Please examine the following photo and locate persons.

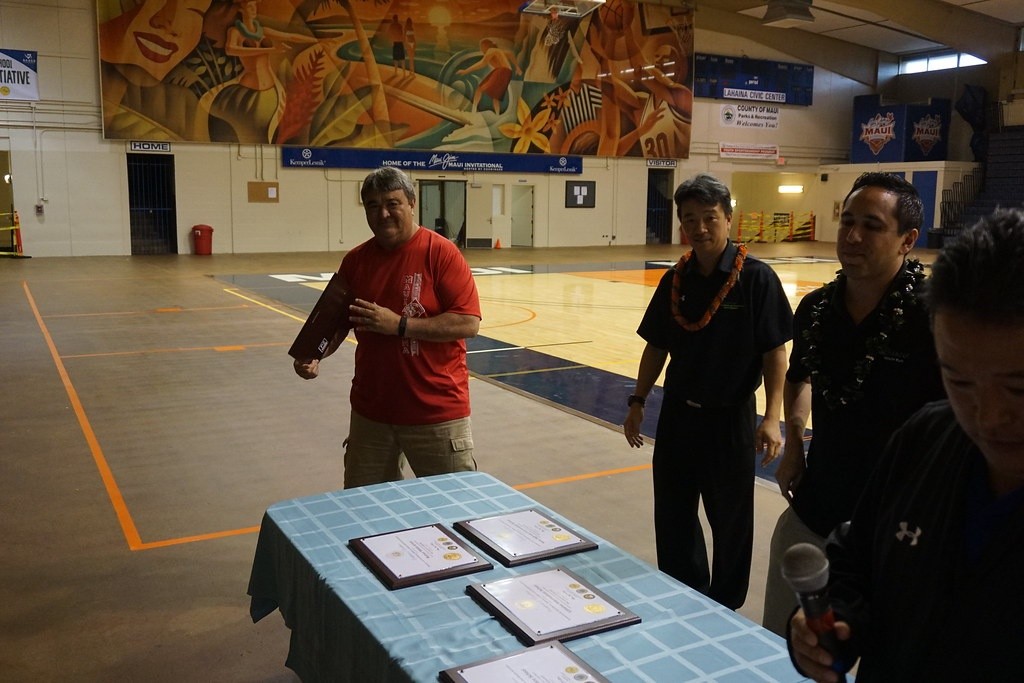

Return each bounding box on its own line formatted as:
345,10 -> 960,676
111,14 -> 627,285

784,207 -> 1024,683
622,175 -> 794,612
293,162 -> 482,488
763,171 -> 946,634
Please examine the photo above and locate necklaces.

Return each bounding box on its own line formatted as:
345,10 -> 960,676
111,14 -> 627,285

671,242 -> 747,331
802,259 -> 926,409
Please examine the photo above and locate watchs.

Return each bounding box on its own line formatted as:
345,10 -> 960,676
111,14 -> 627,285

628,393 -> 645,408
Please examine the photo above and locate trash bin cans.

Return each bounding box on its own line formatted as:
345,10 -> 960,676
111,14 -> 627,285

192,224 -> 213,255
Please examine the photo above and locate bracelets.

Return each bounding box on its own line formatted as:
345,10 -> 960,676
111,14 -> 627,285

399,315 -> 407,337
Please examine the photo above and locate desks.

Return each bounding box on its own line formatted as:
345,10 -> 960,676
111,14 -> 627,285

246,469 -> 856,683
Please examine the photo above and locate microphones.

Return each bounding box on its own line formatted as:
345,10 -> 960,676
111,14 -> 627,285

781,542 -> 847,683
